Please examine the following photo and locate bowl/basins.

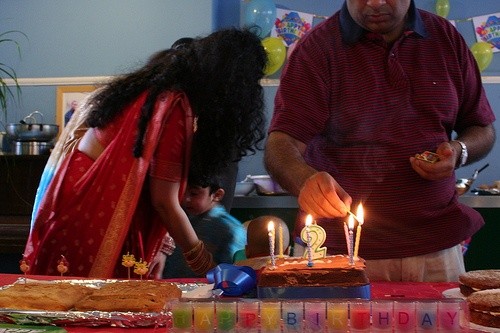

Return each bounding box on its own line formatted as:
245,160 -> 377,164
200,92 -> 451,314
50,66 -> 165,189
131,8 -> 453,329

250,175 -> 287,195
234,182 -> 257,196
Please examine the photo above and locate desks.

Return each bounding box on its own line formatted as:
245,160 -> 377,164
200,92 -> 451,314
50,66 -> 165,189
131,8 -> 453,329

0,272 -> 486,333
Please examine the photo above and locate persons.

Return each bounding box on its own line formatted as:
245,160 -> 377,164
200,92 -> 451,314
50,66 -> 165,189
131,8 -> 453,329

264,0 -> 496,281
64,100 -> 79,127
19,28 -> 264,278
163,138 -> 252,278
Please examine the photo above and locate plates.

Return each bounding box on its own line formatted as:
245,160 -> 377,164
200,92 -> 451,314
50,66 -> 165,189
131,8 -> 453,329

471,188 -> 500,196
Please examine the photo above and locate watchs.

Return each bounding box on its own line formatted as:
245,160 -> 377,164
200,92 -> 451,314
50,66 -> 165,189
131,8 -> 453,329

452,140 -> 468,167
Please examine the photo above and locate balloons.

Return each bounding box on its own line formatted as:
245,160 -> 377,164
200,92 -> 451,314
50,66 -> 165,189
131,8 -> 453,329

241,0 -> 494,77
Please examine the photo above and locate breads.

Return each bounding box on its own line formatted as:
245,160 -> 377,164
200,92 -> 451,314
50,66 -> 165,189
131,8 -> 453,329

75,280 -> 183,313
0,281 -> 95,311
458,268 -> 500,328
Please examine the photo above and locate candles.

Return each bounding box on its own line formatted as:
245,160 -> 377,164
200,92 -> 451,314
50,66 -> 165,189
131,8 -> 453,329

344,201 -> 366,265
268,220 -> 284,267
305,214 -> 314,262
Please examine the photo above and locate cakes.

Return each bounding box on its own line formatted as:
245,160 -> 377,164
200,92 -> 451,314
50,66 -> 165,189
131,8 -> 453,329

256,253 -> 371,301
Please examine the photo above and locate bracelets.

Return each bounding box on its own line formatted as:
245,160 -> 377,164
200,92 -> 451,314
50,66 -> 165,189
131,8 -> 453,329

182,241 -> 213,275
159,234 -> 176,256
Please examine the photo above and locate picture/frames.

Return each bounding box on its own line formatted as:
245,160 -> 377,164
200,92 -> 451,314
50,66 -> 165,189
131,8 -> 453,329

58,84 -> 102,139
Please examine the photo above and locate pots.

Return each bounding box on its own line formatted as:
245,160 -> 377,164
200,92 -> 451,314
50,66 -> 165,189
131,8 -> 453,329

455,169 -> 478,195
6,111 -> 59,141
